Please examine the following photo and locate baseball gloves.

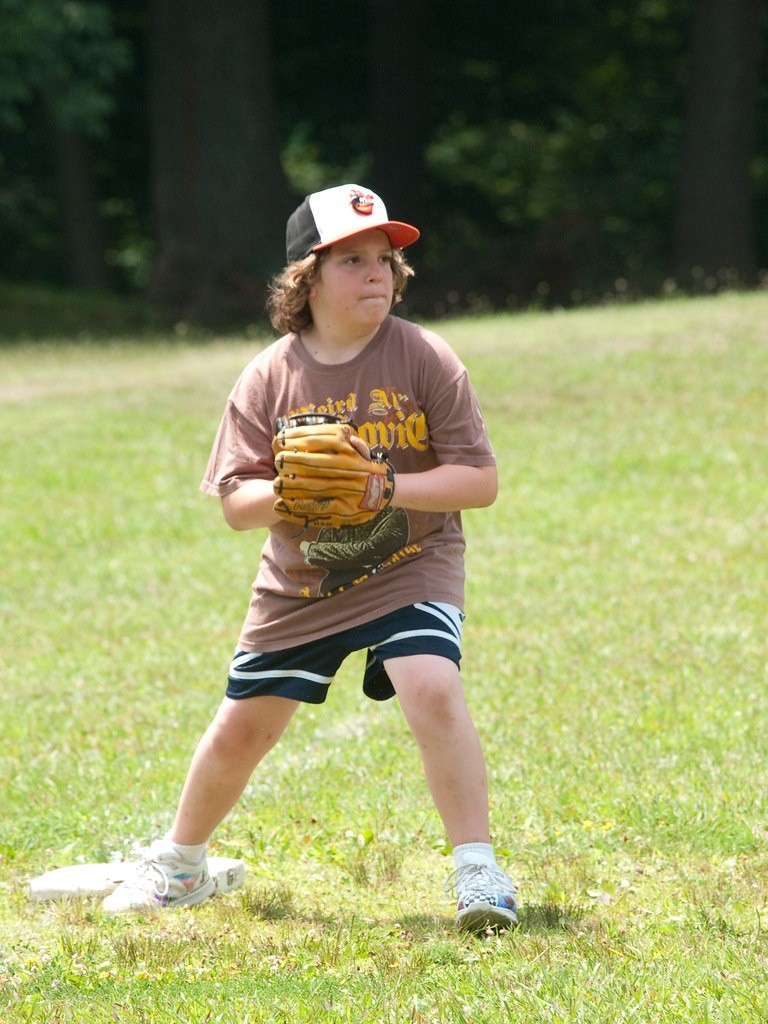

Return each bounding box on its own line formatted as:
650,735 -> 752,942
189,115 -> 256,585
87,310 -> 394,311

270,420 -> 395,530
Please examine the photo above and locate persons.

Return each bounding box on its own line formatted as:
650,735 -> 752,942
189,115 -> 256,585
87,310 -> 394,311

103,184 -> 518,933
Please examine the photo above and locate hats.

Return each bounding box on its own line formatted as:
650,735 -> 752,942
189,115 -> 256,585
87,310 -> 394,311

285,183 -> 421,265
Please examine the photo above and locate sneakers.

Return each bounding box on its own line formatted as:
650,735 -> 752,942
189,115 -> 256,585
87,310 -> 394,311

443,851 -> 518,929
104,841 -> 214,913
